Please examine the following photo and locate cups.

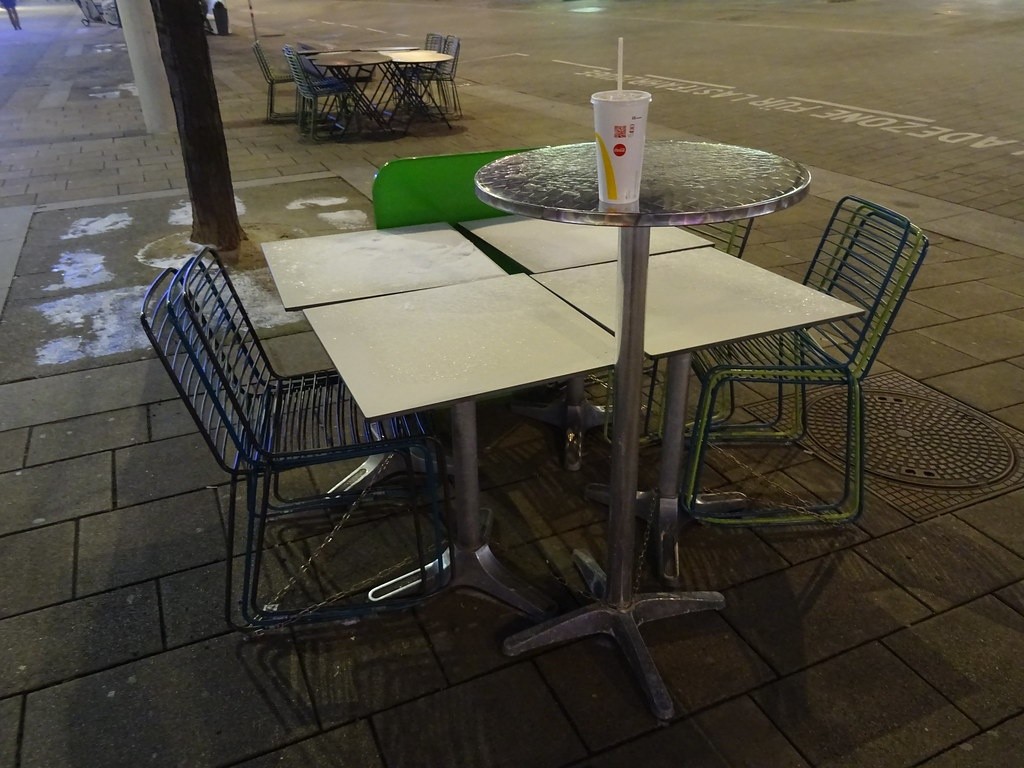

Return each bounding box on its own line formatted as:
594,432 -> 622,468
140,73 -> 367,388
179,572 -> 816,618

591,89 -> 651,203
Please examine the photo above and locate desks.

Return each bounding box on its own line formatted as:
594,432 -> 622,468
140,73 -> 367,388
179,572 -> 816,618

378,50 -> 438,124
474,136 -> 813,723
301,271 -> 649,637
363,46 -> 420,120
259,223 -> 512,518
312,52 -> 398,141
461,205 -> 717,493
304,51 -> 380,136
529,247 -> 866,599
388,52 -> 456,134
296,47 -> 367,120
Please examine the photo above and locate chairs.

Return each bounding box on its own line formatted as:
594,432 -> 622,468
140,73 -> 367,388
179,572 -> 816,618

581,169 -> 930,524
392,31 -> 463,120
251,41 -> 360,141
140,246 -> 456,632
